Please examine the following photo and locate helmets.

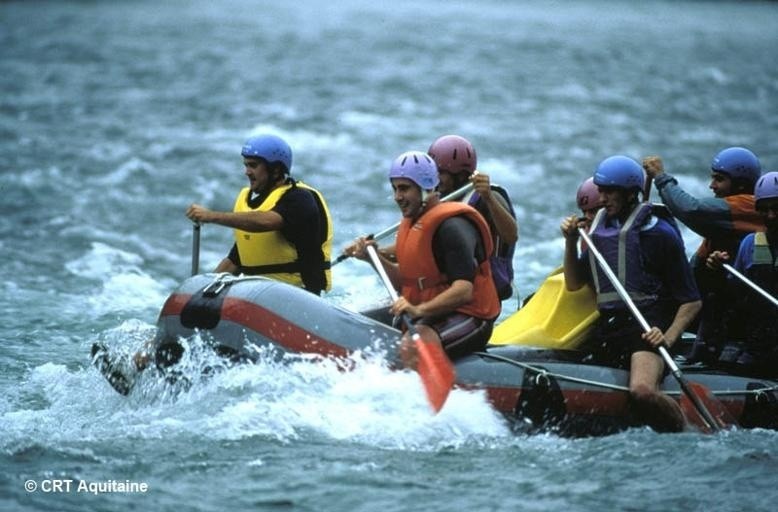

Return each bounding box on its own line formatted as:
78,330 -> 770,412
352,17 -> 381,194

428,134 -> 477,174
711,146 -> 761,183
389,151 -> 442,191
753,173 -> 777,212
576,154 -> 647,211
241,134 -> 291,176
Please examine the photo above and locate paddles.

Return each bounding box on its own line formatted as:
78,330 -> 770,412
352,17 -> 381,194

366,242 -> 456,412
577,226 -> 739,434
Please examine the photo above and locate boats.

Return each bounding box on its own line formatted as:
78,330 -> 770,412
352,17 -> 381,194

155,272 -> 777,437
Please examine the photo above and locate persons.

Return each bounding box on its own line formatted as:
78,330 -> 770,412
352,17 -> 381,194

560,155 -> 702,432
522,177 -> 605,308
643,147 -> 767,334
697,172 -> 778,382
353,151 -> 501,374
91,134 -> 332,394
349,135 -> 518,328
